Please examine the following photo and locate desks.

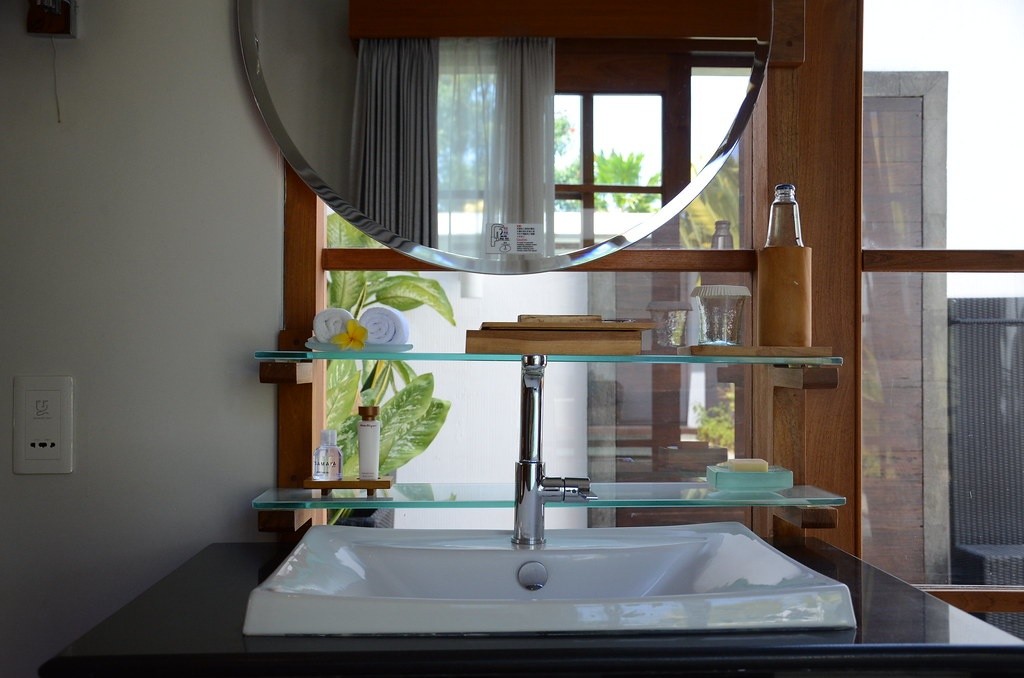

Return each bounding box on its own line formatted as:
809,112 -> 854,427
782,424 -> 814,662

34,544 -> 1024,678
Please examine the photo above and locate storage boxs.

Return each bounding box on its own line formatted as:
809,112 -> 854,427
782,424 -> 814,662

463,330 -> 647,354
706,465 -> 797,490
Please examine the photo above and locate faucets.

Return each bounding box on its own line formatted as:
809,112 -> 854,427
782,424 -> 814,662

510,353 -> 599,546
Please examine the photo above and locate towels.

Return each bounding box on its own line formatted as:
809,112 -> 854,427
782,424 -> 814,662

358,307 -> 406,345
307,307 -> 356,343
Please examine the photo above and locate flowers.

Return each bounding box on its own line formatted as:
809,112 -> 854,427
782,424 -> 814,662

330,318 -> 370,353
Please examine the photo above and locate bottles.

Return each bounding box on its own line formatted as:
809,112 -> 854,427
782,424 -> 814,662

313,430 -> 343,480
764,184 -> 805,247
712,220 -> 734,250
357,406 -> 380,479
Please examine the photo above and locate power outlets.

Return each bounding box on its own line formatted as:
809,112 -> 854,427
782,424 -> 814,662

10,371 -> 77,475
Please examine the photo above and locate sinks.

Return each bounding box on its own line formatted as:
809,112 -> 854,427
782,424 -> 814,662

243,520 -> 857,636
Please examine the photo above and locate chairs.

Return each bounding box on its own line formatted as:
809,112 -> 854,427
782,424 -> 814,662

947,297 -> 1024,639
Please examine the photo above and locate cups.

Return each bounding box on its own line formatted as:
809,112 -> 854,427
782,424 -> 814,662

646,302 -> 694,347
691,285 -> 752,347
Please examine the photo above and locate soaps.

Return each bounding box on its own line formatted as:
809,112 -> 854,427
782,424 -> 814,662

728,458 -> 769,473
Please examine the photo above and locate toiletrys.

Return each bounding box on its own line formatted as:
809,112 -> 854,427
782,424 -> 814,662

357,405 -> 381,481
312,429 -> 344,483
344,481 -> 344,482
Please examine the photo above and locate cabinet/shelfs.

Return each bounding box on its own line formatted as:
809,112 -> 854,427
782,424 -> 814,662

248,338 -> 849,531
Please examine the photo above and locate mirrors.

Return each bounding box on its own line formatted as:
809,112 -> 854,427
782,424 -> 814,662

233,0 -> 774,275
